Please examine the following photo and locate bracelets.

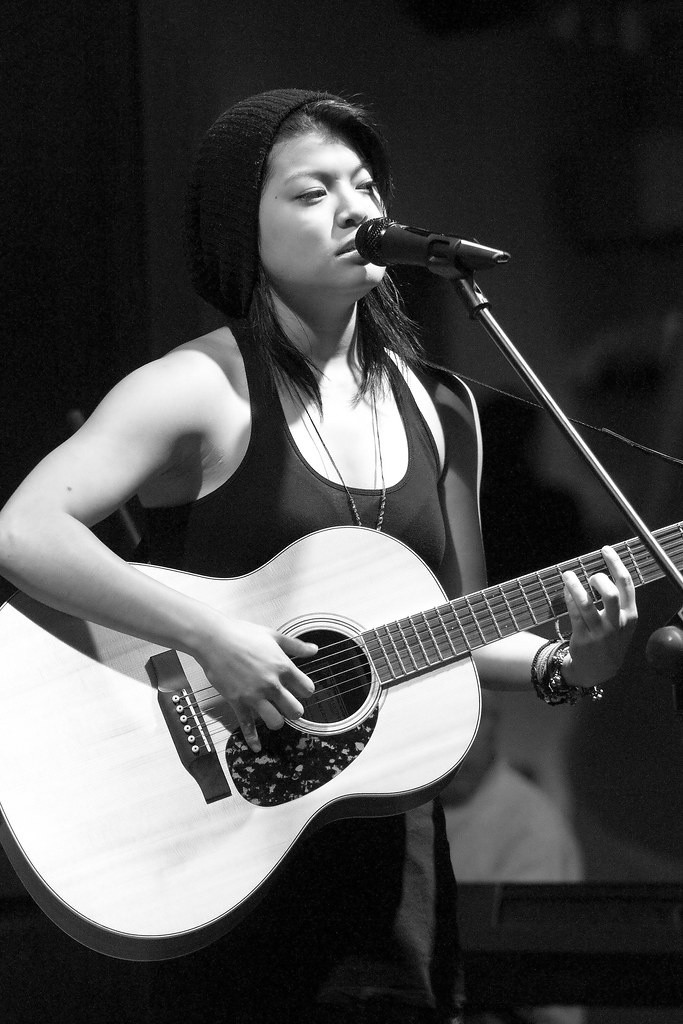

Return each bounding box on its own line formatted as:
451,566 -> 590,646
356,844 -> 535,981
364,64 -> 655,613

530,633 -> 605,707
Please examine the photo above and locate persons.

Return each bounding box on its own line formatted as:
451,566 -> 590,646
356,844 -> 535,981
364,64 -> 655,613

0,86 -> 640,1024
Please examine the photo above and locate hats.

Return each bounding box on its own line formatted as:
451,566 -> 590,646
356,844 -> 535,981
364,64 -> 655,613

179,90 -> 355,319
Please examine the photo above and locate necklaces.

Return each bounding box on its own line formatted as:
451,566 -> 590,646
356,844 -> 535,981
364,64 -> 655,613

288,375 -> 386,533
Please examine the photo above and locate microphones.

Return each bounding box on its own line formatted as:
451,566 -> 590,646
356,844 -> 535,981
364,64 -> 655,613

355,215 -> 510,269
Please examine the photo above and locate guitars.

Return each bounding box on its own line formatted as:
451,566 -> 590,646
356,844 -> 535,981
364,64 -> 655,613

0,523 -> 683,962
